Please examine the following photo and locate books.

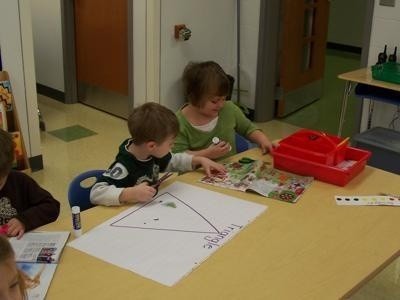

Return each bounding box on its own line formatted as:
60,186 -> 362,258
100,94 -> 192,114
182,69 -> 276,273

9,231 -> 71,300
199,161 -> 315,203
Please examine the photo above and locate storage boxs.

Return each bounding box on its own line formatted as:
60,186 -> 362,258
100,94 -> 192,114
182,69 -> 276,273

352,127 -> 400,172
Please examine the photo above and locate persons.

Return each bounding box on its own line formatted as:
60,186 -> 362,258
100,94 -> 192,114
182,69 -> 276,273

170,61 -> 280,163
90,102 -> 229,206
0,235 -> 29,300
0,128 -> 61,239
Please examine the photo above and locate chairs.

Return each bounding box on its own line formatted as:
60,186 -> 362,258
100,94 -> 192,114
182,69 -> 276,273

67,169 -> 111,213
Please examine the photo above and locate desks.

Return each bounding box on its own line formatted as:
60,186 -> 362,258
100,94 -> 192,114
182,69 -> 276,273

1,138 -> 399,300
334,66 -> 400,138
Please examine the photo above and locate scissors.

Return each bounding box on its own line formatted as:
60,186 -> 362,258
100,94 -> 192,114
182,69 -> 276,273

239,156 -> 273,166
310,128 -> 329,139
141,171 -> 173,198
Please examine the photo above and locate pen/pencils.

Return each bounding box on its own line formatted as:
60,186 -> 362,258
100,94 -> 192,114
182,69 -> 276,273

337,136 -> 350,147
